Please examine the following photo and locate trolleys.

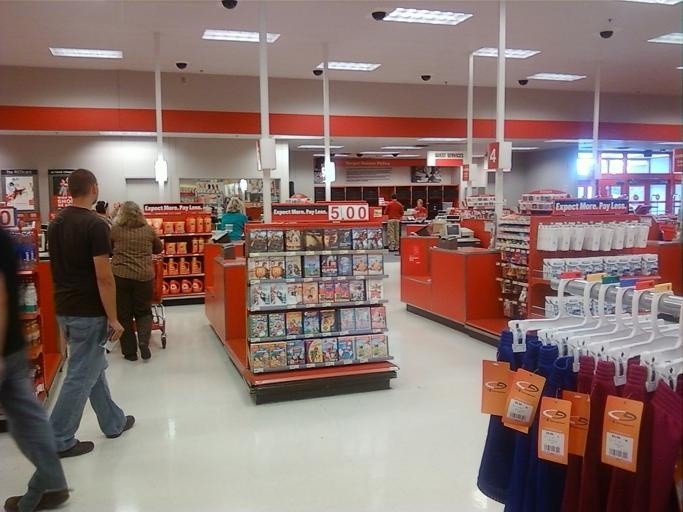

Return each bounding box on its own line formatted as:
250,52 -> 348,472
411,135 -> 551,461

108,252 -> 167,350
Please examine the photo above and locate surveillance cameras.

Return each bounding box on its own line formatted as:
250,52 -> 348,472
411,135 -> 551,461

598,18 -> 614,38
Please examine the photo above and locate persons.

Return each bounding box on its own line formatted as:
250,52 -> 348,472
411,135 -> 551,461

95,200 -> 114,258
412,199 -> 429,219
110,200 -> 164,360
416,166 -> 443,183
0,223 -> 72,512
46,168 -> 135,459
221,197 -> 248,241
384,194 -> 404,252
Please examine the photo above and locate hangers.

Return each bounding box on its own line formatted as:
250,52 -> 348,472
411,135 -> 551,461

508,278 -> 683,392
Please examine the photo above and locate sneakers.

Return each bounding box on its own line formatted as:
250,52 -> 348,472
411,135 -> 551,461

125,354 -> 138,361
139,342 -> 151,359
58,440 -> 95,458
106,415 -> 136,438
4,489 -> 71,510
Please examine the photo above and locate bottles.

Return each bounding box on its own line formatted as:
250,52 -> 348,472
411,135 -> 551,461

185,215 -> 212,255
16,245 -> 47,403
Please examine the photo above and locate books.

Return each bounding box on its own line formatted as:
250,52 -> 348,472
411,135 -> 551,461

247,228 -> 389,370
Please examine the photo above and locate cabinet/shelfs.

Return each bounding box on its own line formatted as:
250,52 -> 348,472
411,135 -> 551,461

134,212 -> 216,300
1,260 -> 68,432
245,224 -> 399,387
495,212 -> 641,318
